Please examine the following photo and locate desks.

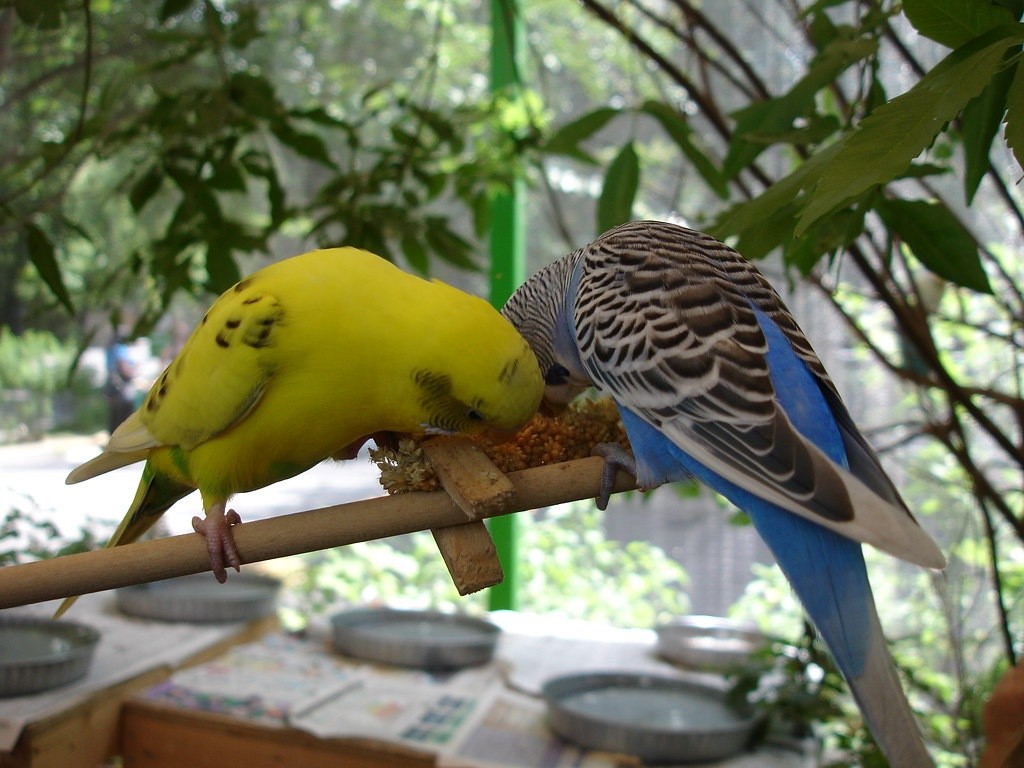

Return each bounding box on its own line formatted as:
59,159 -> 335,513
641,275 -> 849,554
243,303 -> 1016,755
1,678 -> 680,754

1,611 -> 439,768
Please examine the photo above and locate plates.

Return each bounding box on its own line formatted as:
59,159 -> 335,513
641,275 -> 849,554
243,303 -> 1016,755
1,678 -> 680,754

114,574 -> 284,622
538,671 -> 766,761
0,608 -> 100,697
651,618 -> 775,671
331,610 -> 501,670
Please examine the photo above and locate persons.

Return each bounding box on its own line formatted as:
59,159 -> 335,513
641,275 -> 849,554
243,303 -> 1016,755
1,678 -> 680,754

106,340 -> 136,437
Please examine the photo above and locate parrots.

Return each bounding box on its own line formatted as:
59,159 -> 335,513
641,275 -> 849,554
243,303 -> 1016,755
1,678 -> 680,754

56,219 -> 949,767
